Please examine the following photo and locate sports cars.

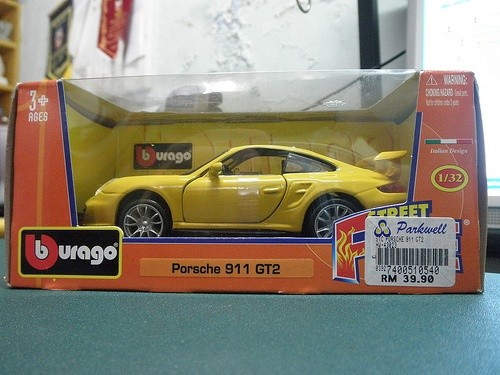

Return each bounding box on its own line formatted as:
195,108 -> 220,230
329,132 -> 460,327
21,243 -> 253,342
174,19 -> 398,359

83,144 -> 408,237
